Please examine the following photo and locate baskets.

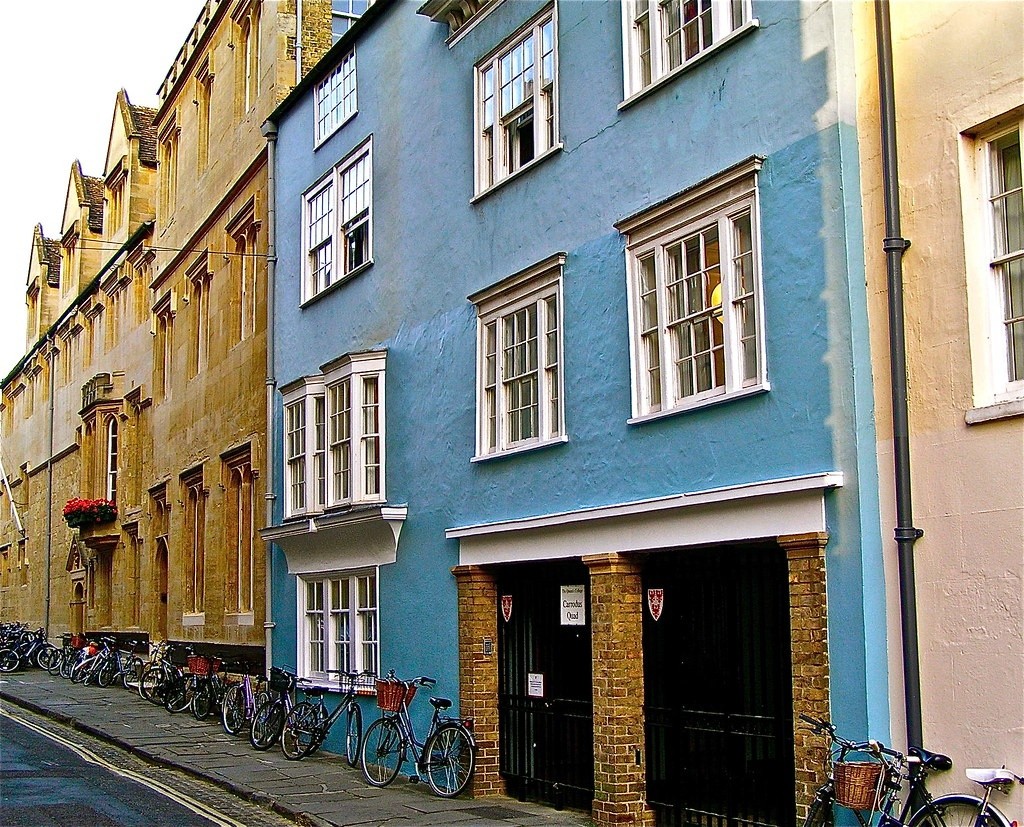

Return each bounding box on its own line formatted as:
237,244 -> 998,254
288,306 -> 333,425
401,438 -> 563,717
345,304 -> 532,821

372,678 -> 418,713
187,655 -> 223,676
71,635 -> 87,649
268,666 -> 297,693
834,758 -> 892,811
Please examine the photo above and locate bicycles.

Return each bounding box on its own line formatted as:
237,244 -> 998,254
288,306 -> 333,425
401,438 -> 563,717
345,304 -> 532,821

0,620 -> 273,735
798,712 -> 1018,827
869,739 -> 1024,827
361,670 -> 479,798
281,669 -> 378,766
248,665 -> 329,755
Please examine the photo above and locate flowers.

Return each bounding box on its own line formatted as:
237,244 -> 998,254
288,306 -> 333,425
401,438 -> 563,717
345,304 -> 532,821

62,497 -> 118,528
